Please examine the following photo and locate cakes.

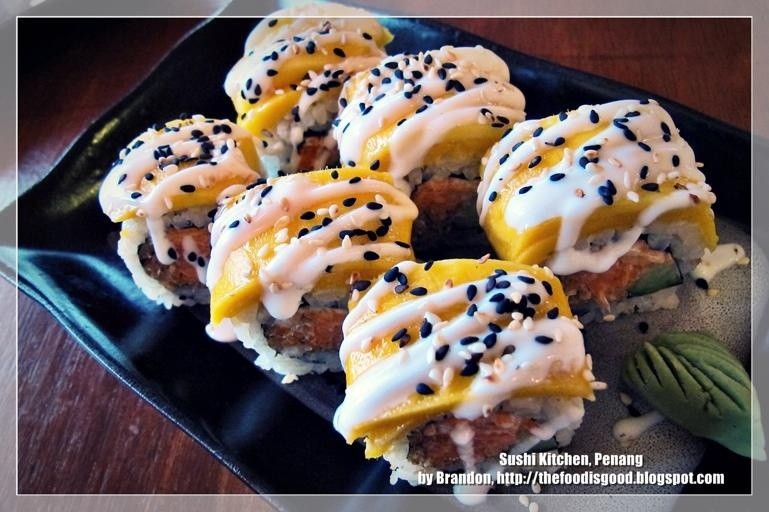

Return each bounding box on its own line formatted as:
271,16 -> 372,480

98,3 -> 718,506
